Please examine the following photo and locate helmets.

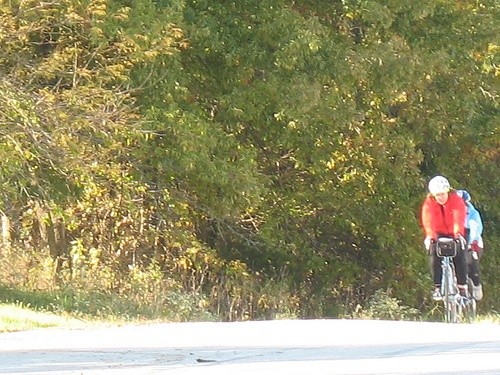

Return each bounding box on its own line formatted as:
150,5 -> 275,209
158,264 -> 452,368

457,190 -> 472,201
428,176 -> 450,194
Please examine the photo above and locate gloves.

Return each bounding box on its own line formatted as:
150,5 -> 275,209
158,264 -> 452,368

455,233 -> 466,250
424,237 -> 433,250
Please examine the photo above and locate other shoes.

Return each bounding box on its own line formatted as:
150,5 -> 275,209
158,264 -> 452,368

470,280 -> 483,301
432,288 -> 442,301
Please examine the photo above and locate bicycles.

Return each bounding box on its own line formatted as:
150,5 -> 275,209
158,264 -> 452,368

427,237 -> 477,324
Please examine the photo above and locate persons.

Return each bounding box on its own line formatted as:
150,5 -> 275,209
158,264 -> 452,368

422,175 -> 469,306
456,190 -> 483,300
